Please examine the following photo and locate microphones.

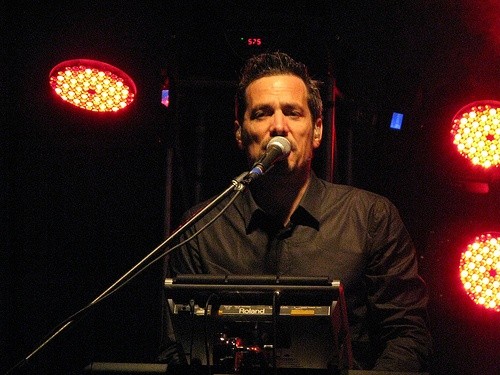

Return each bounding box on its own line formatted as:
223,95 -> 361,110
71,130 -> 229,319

241,136 -> 290,186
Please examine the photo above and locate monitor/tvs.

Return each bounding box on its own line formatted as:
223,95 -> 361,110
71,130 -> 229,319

162,274 -> 354,371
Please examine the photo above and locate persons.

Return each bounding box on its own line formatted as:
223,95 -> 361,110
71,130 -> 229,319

159,49 -> 432,374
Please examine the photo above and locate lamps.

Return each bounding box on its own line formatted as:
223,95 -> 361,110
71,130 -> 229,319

450,99 -> 500,169
49,57 -> 137,113
459,230 -> 499,313
